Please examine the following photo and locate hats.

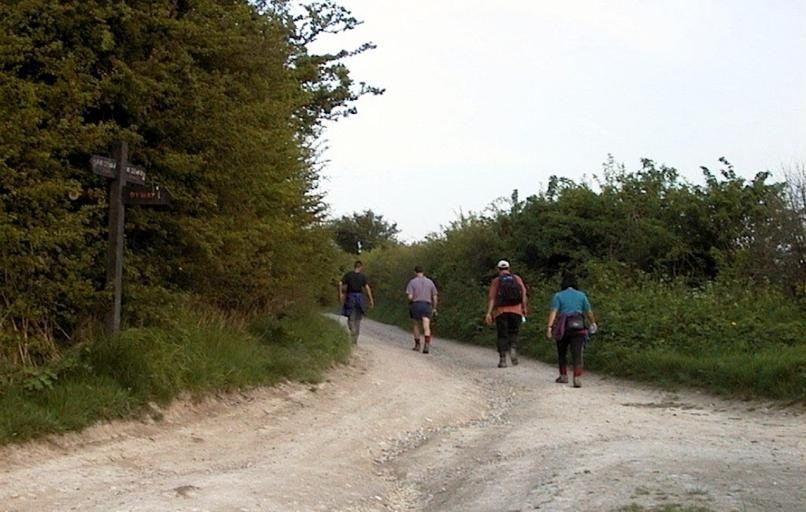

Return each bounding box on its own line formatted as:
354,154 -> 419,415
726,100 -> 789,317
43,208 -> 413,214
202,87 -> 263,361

497,260 -> 510,268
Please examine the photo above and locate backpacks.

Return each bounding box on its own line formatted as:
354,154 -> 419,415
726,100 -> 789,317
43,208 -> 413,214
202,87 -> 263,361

496,274 -> 523,307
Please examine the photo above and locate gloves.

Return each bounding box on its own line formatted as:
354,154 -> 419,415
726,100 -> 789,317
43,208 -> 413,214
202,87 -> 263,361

589,323 -> 597,334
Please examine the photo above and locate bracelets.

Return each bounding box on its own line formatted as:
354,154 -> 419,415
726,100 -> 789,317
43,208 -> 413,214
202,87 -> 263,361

548,325 -> 552,328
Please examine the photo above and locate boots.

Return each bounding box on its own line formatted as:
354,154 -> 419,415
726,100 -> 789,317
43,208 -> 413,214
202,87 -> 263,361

498,350 -> 518,367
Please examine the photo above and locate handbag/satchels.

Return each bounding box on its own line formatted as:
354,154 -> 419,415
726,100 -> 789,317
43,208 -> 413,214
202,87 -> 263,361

567,314 -> 588,330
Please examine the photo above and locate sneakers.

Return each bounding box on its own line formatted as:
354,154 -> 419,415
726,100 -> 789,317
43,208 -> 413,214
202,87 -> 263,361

412,344 -> 429,353
556,375 -> 568,383
574,376 -> 581,387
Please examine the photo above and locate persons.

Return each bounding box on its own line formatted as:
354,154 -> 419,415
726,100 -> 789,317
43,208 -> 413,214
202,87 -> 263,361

546,272 -> 597,387
485,259 -> 527,368
338,260 -> 373,345
406,265 -> 438,353
